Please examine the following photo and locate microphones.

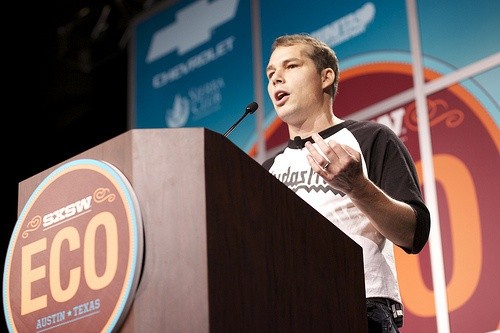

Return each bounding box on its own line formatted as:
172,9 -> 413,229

294,135 -> 302,151
223,102 -> 259,138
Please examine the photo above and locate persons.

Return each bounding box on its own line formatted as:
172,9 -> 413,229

259,33 -> 432,333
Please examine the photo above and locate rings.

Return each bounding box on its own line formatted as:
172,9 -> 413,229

323,161 -> 331,171
